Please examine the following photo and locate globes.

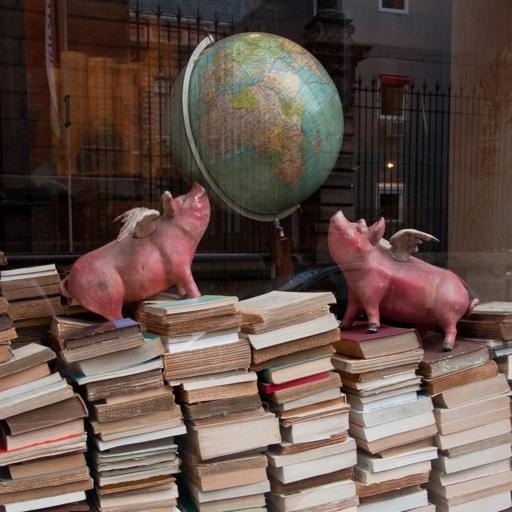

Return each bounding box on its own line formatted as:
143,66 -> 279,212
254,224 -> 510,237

164,30 -> 359,287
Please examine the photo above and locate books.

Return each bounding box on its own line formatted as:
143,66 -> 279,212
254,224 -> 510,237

1,264 -> 94,512
415,300 -> 512,512
133,292 -> 281,512
49,314 -> 188,512
331,319 -> 438,512
238,291 -> 360,512
